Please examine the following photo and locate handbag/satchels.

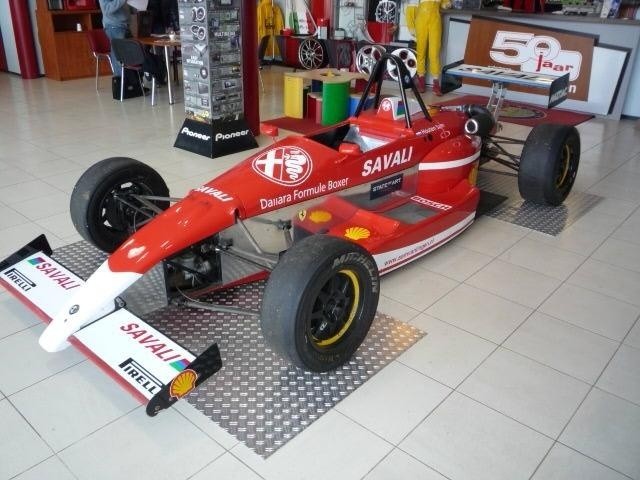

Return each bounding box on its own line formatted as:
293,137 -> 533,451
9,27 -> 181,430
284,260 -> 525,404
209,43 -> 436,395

112,76 -> 140,99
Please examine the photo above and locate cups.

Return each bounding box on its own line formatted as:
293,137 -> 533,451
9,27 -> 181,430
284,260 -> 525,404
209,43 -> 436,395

76,23 -> 83,32
168,34 -> 176,41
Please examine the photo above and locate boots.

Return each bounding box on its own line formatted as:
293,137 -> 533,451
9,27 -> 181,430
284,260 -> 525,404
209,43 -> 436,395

416,76 -> 426,92
432,80 -> 442,97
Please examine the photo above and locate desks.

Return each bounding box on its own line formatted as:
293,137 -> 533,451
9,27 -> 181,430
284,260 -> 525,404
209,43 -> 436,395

284,68 -> 377,126
134,36 -> 181,106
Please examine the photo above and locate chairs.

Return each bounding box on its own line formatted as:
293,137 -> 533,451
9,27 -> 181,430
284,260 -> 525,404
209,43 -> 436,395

111,38 -> 162,106
87,29 -> 115,90
256,33 -> 271,92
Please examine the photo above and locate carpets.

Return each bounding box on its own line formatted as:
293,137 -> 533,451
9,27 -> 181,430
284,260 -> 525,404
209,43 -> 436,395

427,94 -> 595,129
474,159 -> 604,235
51,240 -> 426,459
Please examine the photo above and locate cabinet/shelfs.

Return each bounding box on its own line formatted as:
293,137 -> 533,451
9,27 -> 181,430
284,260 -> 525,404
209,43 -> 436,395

35,0 -> 104,81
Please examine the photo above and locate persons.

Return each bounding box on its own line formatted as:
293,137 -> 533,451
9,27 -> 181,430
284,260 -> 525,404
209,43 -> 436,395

98,0 -> 138,77
404,0 -> 454,96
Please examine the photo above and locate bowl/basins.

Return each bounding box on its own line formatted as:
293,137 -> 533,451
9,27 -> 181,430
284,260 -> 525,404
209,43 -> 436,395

280,28 -> 295,37
